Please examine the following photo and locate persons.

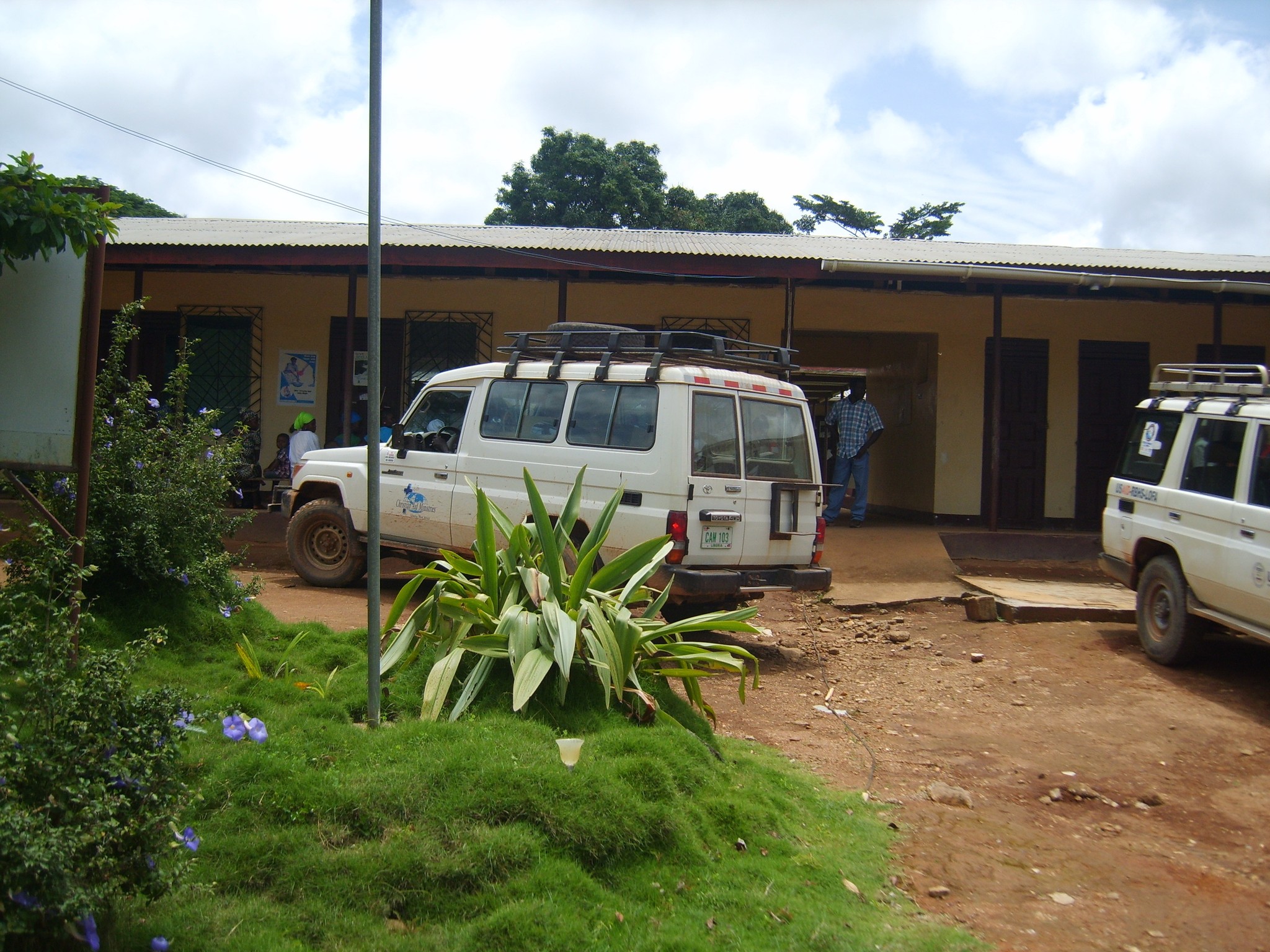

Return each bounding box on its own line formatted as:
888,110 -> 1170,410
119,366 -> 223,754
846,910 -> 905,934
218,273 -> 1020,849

225,405 -> 454,511
818,377 -> 884,528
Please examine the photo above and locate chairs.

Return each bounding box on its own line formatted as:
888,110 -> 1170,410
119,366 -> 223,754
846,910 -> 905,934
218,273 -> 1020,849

1194,440 -> 1241,497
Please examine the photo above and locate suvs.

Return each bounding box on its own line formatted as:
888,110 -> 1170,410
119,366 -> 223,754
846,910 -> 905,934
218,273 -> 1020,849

1097,361 -> 1270,667
266,319 -> 832,638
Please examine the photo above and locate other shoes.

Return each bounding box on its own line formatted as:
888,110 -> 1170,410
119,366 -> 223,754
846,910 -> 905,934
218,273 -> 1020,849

825,520 -> 835,526
230,504 -> 239,508
266,496 -> 271,500
849,520 -> 862,527
253,505 -> 266,509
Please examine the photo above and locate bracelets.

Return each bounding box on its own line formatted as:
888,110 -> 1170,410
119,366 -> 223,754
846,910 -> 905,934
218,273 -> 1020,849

864,444 -> 869,449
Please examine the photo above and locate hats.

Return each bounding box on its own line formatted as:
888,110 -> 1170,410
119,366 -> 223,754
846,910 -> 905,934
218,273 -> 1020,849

427,419 -> 445,432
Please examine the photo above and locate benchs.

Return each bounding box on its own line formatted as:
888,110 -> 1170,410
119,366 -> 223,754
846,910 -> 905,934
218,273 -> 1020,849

228,477 -> 292,486
